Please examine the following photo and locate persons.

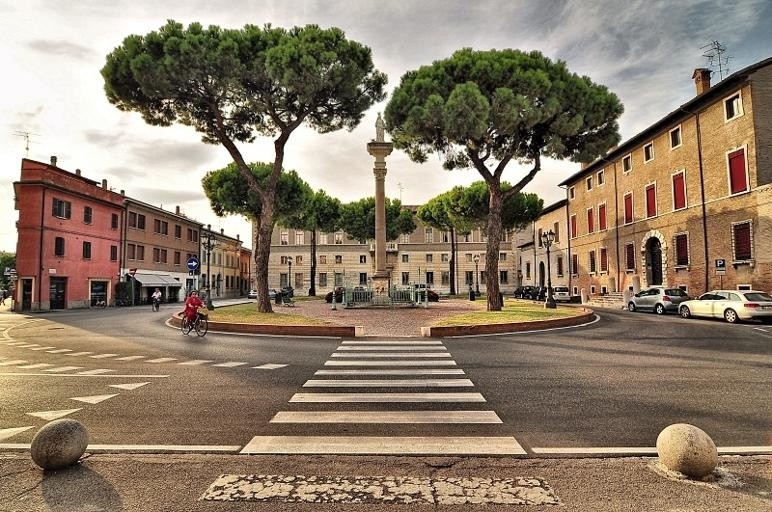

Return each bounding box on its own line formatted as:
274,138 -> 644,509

151,287 -> 161,307
183,290 -> 203,333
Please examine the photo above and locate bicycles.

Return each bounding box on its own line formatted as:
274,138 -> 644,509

181,306 -> 209,337
151,295 -> 160,312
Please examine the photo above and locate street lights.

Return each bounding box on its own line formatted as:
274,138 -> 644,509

200,225 -> 215,310
541,229 -> 556,308
472,251 -> 480,297
287,256 -> 292,287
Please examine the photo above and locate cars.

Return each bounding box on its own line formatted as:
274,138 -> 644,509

514,285 -> 571,302
248,288 -> 278,298
628,287 -> 690,315
677,289 -> 771,322
